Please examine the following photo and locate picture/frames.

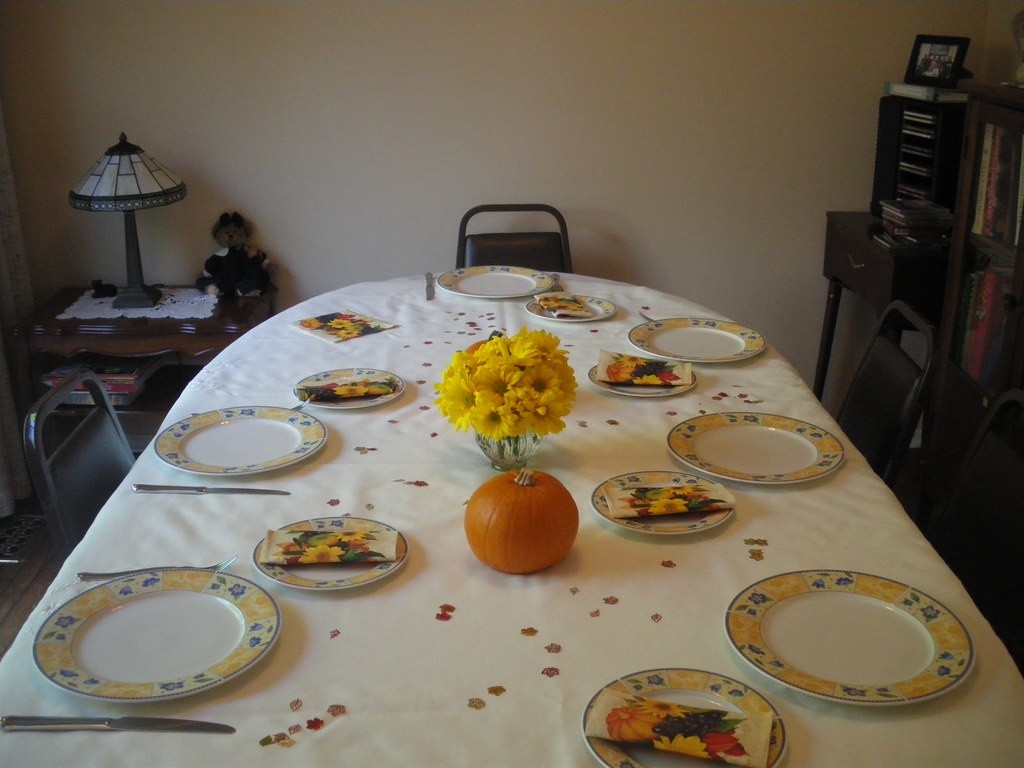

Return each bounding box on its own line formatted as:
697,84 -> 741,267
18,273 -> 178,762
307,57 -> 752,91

904,34 -> 971,89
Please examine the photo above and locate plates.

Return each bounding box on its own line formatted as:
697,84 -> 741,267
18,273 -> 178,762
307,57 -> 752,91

627,318 -> 767,362
725,570 -> 974,705
580,667 -> 788,768
153,406 -> 328,475
588,365 -> 697,397
666,412 -> 845,484
31,567 -> 281,703
293,368 -> 405,408
526,295 -> 615,321
251,517 -> 409,591
436,265 -> 554,298
589,470 -> 734,534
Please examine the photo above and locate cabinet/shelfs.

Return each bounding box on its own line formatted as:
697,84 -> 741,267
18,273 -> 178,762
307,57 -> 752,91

937,76 -> 1023,405
8,285 -> 273,485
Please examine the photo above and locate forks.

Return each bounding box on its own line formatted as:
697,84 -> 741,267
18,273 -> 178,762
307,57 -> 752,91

551,274 -> 563,292
190,394 -> 315,415
638,311 -> 653,321
76,553 -> 237,582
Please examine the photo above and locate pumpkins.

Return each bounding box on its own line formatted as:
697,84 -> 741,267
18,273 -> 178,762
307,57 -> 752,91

465,330 -> 503,357
464,468 -> 580,574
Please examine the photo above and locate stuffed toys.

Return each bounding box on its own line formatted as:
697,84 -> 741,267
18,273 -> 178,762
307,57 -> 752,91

194,212 -> 271,306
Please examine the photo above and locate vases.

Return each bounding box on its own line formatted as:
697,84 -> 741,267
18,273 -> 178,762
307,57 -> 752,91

475,424 -> 543,475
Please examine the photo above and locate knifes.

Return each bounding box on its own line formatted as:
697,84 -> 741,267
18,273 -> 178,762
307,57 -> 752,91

424,272 -> 435,300
0,715 -> 237,734
131,483 -> 291,496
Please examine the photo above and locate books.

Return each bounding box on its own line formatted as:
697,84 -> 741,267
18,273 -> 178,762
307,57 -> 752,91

40,355 -> 163,405
873,124 -> 1024,379
884,82 -> 969,104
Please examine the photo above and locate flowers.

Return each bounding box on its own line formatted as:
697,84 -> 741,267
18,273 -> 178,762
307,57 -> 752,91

436,330 -> 577,441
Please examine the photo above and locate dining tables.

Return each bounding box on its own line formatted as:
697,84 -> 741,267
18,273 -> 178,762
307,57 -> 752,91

0,266 -> 1024,768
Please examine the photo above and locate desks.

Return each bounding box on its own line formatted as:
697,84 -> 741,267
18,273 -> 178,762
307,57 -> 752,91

811,212 -> 951,401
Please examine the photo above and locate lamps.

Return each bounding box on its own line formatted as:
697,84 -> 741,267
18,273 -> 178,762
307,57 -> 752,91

68,132 -> 187,309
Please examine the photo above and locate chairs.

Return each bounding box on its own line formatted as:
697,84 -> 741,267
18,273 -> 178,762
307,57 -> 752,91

22,368 -> 139,564
929,388 -> 1024,652
456,204 -> 573,279
835,299 -> 939,487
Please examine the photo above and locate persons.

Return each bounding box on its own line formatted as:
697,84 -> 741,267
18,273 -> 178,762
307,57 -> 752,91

920,55 -> 954,79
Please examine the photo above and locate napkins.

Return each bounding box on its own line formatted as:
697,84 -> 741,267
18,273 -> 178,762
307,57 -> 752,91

291,309 -> 398,344
533,292 -> 592,318
584,686 -> 774,768
601,482 -> 736,519
259,526 -> 399,563
595,349 -> 692,387
294,378 -> 396,404
